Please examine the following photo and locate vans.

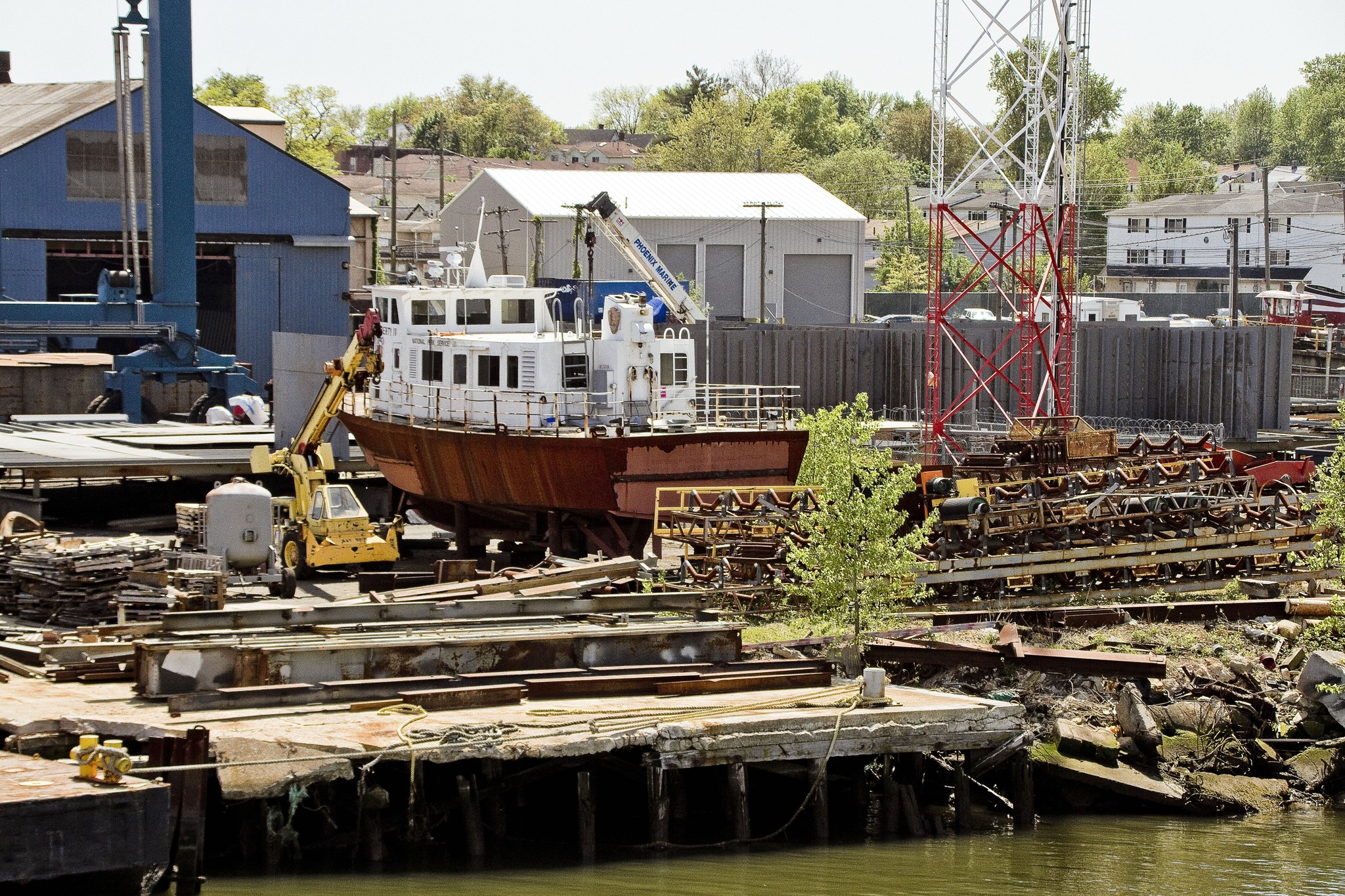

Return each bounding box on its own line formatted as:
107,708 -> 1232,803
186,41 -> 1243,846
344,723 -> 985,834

961,308 -> 1030,322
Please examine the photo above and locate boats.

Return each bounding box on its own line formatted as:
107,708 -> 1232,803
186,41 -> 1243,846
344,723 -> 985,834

337,110 -> 809,519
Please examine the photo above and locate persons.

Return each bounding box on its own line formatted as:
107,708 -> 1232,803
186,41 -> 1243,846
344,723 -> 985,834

264,378 -> 273,423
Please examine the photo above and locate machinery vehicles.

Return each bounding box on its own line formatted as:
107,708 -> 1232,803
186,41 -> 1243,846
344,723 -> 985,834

250,307 -> 405,598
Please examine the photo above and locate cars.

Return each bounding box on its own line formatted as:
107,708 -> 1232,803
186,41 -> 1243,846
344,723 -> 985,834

920,307 -> 938,316
1140,312 -> 1215,327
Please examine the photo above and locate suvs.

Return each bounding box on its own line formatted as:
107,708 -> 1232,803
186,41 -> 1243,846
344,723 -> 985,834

1211,308 -> 1248,326
871,315 -> 927,324
